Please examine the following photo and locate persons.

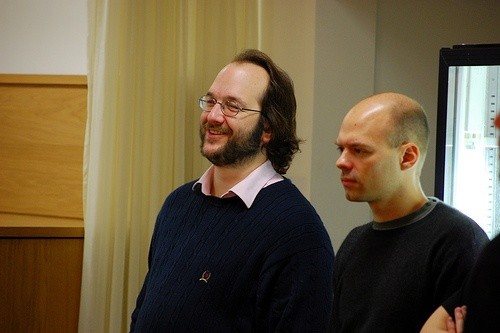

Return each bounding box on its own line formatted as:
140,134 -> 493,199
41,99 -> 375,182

331,93 -> 491,333
418,110 -> 500,333
123,46 -> 340,333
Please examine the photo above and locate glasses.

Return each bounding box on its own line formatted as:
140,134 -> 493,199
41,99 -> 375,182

198,95 -> 264,117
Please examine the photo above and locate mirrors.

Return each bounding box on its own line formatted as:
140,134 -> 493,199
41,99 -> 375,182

433,43 -> 500,241
